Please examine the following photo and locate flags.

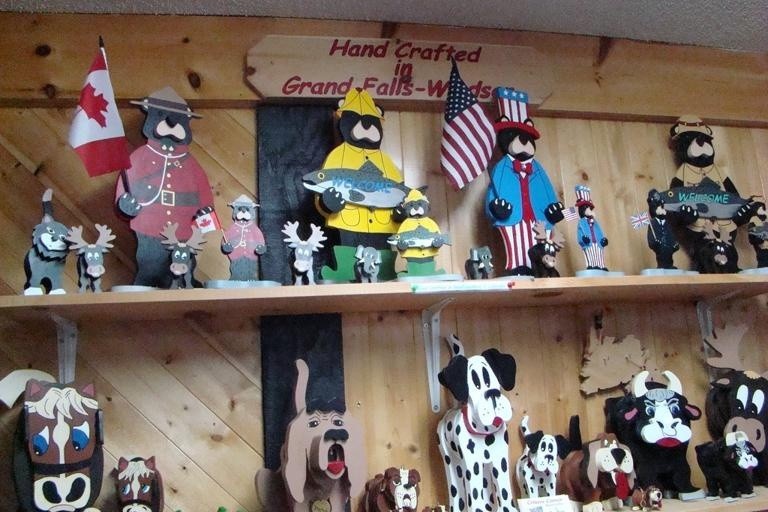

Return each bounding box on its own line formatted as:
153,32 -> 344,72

67,43 -> 132,177
438,60 -> 501,192
